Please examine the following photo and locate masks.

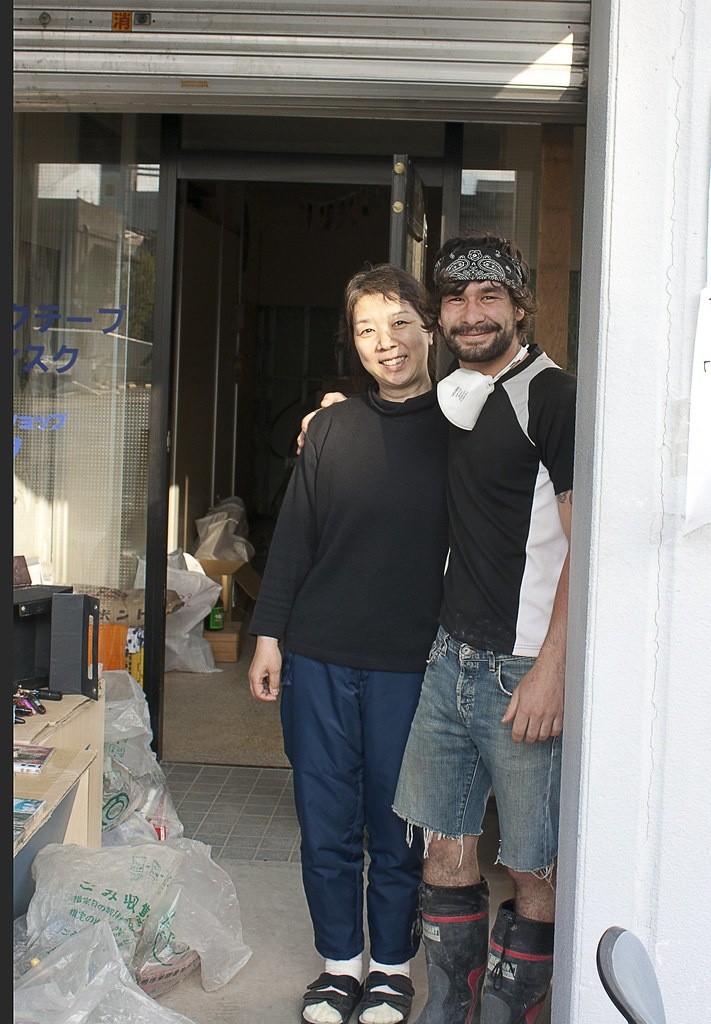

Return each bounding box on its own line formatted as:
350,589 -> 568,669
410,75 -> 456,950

437,344 -> 529,430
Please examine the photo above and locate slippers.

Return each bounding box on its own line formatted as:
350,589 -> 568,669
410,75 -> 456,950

300,972 -> 365,1024
356,970 -> 415,1024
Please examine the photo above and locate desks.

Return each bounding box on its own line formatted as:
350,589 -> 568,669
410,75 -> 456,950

13,680 -> 107,916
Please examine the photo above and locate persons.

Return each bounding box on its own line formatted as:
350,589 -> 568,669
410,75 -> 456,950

297,232 -> 577,1024
248,263 -> 449,1024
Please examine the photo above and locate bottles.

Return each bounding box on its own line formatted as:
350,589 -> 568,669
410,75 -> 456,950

205,596 -> 224,632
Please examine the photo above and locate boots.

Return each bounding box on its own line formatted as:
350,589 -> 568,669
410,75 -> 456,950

413,875 -> 491,1024
479,898 -> 555,1024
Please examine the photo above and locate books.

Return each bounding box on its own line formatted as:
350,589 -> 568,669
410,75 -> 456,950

13,742 -> 56,775
13,796 -> 48,853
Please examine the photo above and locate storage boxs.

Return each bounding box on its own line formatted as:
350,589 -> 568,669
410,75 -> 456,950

203,621 -> 242,663
